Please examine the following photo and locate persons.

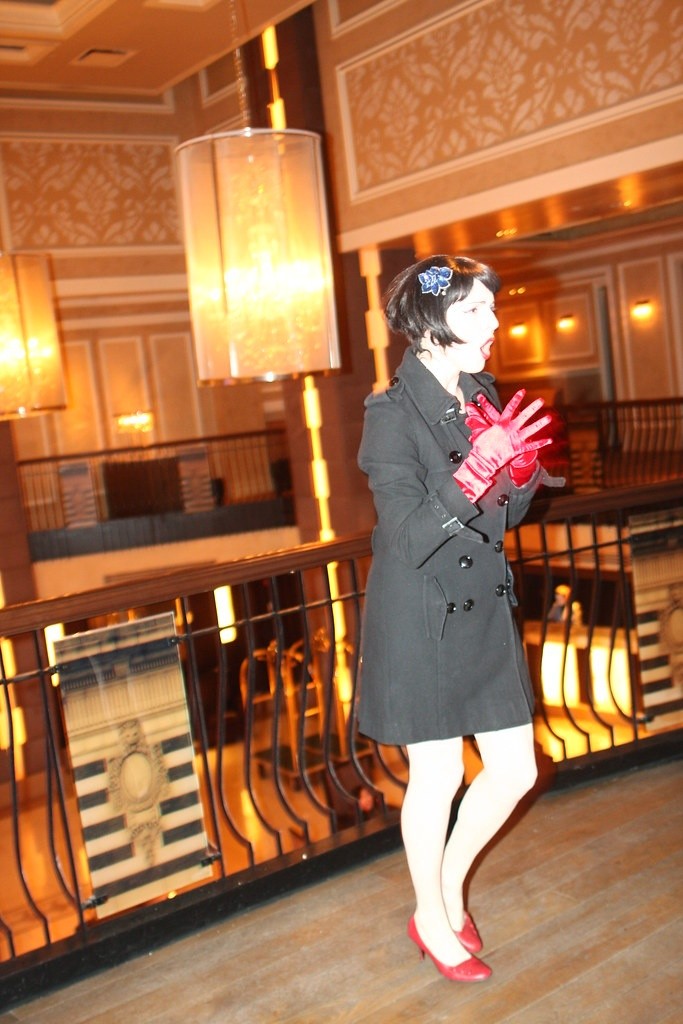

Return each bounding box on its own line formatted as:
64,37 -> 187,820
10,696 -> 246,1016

355,254 -> 554,984
547,584 -> 583,623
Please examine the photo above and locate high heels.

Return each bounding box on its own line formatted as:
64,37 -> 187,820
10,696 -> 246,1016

407,914 -> 491,983
450,910 -> 484,953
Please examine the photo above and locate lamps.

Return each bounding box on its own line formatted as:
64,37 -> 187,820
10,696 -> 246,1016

0,253 -> 68,423
172,0 -> 340,381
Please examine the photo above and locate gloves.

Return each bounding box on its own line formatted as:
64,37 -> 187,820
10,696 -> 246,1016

462,393 -> 538,488
453,389 -> 554,503
